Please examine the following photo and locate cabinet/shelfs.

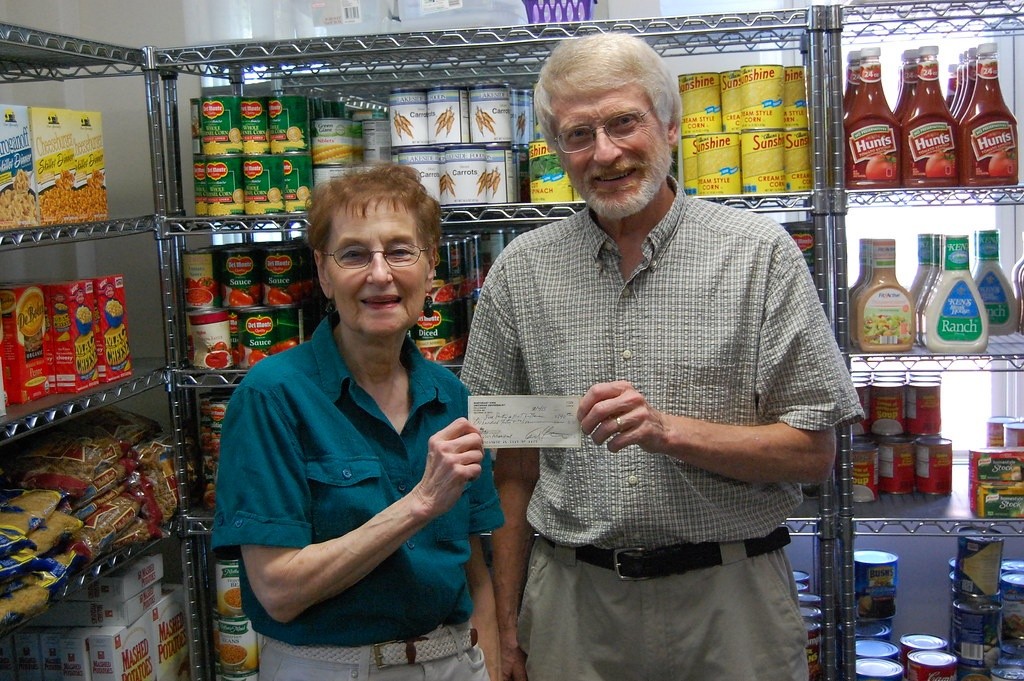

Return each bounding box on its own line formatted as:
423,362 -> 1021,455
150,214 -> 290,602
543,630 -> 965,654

0,17 -> 205,681
830,1 -> 1024,681
155,9 -> 840,681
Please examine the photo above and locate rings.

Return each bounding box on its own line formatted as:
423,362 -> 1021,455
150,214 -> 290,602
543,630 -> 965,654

616,416 -> 620,425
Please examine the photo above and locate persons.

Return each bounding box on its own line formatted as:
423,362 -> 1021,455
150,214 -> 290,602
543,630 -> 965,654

459,34 -> 866,681
212,168 -> 500,681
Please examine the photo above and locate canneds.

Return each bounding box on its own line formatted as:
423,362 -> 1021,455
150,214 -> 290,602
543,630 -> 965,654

780,221 -> 815,281
675,64 -> 812,196
187,80 -> 589,218
793,569 -> 823,681
179,228 -> 536,372
199,386 -> 229,513
850,372 -> 953,503
854,526 -> 1024,681
208,546 -> 262,681
986,416 -> 1024,447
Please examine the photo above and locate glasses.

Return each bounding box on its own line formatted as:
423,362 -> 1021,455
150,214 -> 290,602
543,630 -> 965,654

319,241 -> 431,269
552,104 -> 654,154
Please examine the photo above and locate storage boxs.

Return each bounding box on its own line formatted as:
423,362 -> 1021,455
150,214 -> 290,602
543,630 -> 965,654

0,104 -> 109,231
0,548 -> 189,681
0,275 -> 132,418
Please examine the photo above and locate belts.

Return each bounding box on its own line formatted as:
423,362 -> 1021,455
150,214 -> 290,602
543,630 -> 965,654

264,618 -> 480,670
540,524 -> 792,581
